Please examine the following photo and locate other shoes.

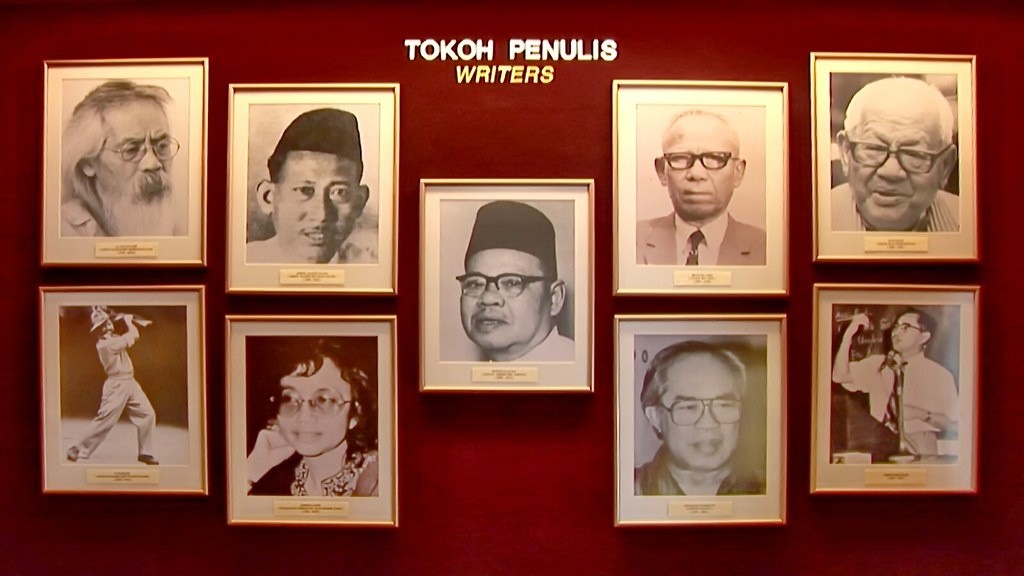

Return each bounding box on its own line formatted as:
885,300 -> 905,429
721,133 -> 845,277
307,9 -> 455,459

138,454 -> 159,465
68,446 -> 79,460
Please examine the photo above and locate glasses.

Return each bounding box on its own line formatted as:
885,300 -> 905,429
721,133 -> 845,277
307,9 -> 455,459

662,150 -> 739,170
456,271 -> 552,298
659,396 -> 742,425
102,137 -> 180,161
892,321 -> 923,334
845,131 -> 951,174
269,386 -> 355,417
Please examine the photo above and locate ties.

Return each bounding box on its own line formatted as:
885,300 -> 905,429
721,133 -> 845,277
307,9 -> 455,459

684,230 -> 706,266
882,358 -> 908,434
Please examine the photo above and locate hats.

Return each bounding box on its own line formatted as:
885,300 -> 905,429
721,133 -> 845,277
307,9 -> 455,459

276,108 -> 361,163
464,201 -> 557,279
90,308 -> 113,332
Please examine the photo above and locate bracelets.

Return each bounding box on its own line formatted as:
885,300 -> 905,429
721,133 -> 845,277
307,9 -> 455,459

247,477 -> 256,488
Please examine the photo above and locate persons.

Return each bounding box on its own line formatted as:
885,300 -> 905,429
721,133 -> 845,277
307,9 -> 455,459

66,305 -> 159,466
455,200 -> 573,362
635,110 -> 767,266
634,341 -> 766,496
832,307 -> 959,456
247,338 -> 378,497
831,75 -> 959,232
60,80 -> 189,237
246,107 -> 379,265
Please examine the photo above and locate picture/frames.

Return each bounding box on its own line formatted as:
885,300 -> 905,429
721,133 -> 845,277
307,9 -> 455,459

41,57 -> 207,266
39,284 -> 208,495
223,316 -> 399,526
224,83 -> 400,296
611,77 -> 789,296
810,51 -> 982,261
809,283 -> 980,494
419,178 -> 595,393
612,311 -> 787,524
